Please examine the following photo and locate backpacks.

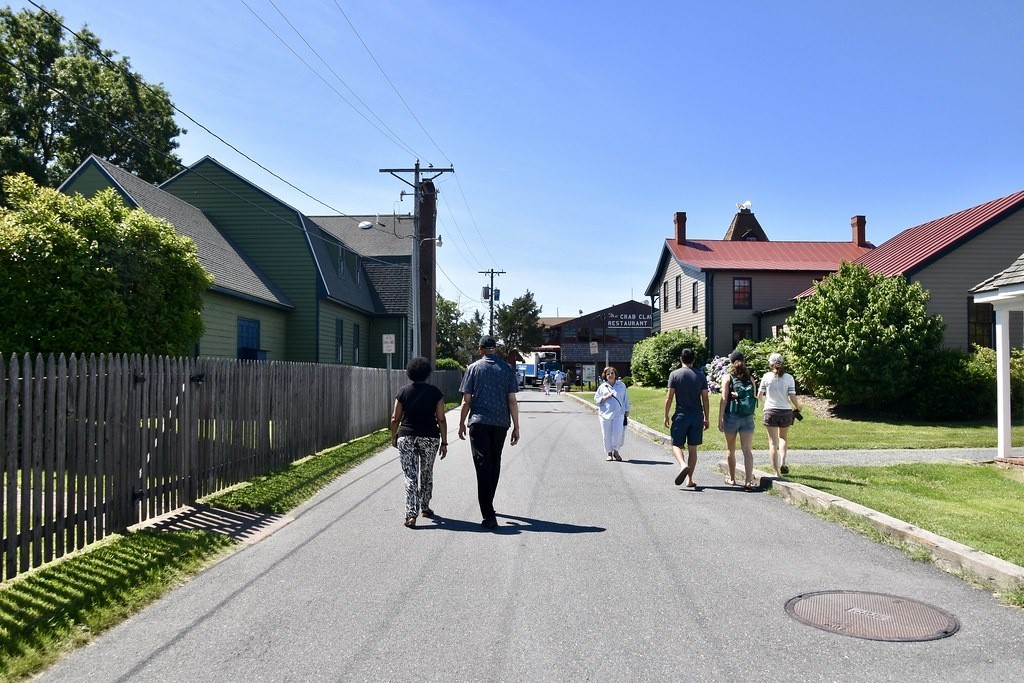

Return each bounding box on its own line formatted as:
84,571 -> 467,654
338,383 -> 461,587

729,372 -> 758,416
546,375 -> 552,383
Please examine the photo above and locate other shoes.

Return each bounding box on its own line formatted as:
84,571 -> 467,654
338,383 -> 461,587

482,519 -> 498,530
606,452 -> 613,461
781,465 -> 789,474
404,517 -> 416,527
613,450 -> 622,461
422,508 -> 434,516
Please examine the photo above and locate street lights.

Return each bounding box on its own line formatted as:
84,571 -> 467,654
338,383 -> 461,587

415,234 -> 444,358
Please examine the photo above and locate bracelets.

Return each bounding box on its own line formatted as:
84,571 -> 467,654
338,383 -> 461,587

441,441 -> 448,446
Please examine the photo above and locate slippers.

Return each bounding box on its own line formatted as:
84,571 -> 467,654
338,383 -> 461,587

686,483 -> 696,487
745,482 -> 751,488
724,478 -> 735,485
675,467 -> 690,485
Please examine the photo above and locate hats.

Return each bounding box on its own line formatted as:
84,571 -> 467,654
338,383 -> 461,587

730,351 -> 744,363
768,353 -> 783,364
479,335 -> 496,349
556,370 -> 560,373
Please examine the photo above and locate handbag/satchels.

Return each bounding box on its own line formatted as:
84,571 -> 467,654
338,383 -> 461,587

623,414 -> 627,426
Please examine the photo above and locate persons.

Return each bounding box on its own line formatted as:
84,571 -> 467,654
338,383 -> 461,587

457,335 -> 520,527
757,353 -> 801,478
543,369 -> 552,395
664,349 -> 710,486
391,357 -> 448,526
554,370 -> 562,396
718,351 -> 758,485
565,370 -> 571,388
594,366 -> 630,460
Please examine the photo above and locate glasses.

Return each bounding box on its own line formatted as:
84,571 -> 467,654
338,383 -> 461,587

606,372 -> 615,375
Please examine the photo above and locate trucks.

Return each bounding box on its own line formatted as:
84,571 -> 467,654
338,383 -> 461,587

516,351 -> 567,388
